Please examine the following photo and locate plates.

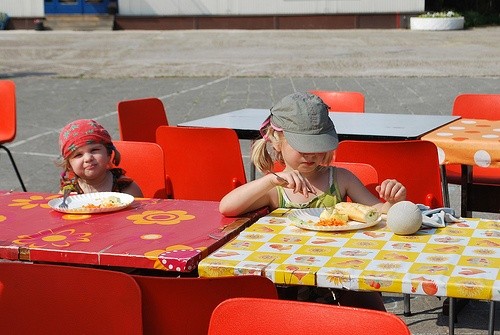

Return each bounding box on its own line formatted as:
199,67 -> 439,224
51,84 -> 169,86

48,192 -> 134,213
285,208 -> 384,231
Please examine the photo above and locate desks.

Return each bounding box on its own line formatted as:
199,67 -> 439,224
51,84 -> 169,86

198,207 -> 500,335
0,189 -> 271,271
177,108 -> 500,206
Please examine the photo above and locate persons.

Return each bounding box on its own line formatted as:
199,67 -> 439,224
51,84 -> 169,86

58,119 -> 145,198
219,92 -> 406,314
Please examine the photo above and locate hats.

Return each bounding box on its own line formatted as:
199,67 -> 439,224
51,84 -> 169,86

269,92 -> 338,154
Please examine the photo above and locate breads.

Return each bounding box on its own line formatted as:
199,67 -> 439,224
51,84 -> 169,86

320,208 -> 348,223
335,201 -> 378,222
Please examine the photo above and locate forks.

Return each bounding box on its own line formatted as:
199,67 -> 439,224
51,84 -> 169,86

58,188 -> 71,209
266,169 -> 329,213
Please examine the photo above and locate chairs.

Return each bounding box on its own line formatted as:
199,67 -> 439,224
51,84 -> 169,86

311,90 -> 500,314
156,126 -> 247,200
0,261 -> 143,335
129,274 -> 278,335
0,78 -> 28,192
210,297 -> 411,335
117,97 -> 169,143
112,140 -> 167,199
